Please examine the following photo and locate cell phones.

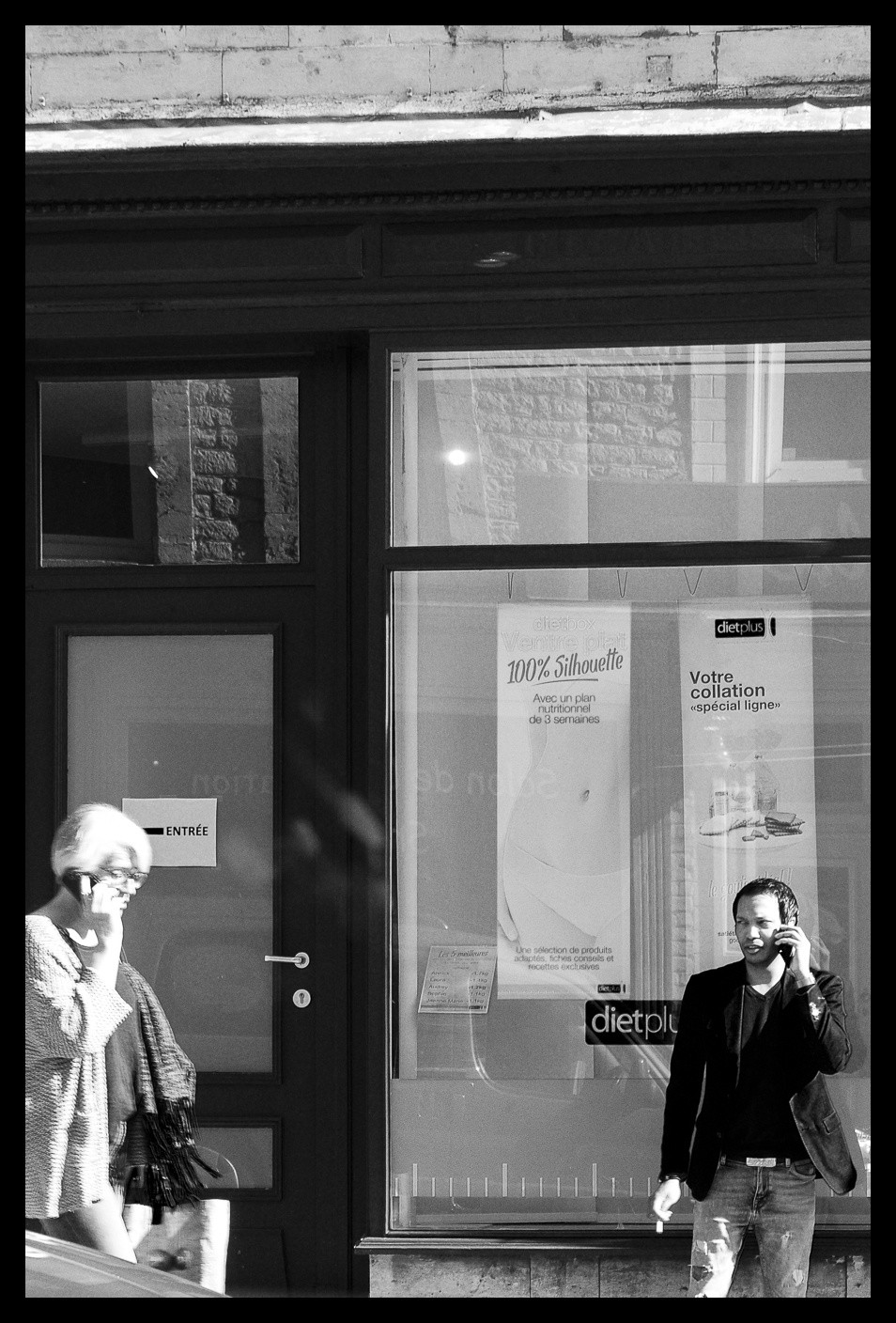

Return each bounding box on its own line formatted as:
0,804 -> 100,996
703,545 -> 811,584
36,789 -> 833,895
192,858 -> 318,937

778,922 -> 797,966
61,867 -> 101,902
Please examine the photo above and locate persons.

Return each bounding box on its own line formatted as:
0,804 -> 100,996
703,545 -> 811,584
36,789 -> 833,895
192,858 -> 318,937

653,877 -> 860,1298
26,803 -> 153,1264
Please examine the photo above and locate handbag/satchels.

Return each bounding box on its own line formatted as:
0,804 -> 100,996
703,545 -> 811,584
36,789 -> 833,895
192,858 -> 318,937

121,1107 -> 232,1294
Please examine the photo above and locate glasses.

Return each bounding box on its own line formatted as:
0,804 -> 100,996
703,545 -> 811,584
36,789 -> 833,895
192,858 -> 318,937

98,867 -> 149,890
